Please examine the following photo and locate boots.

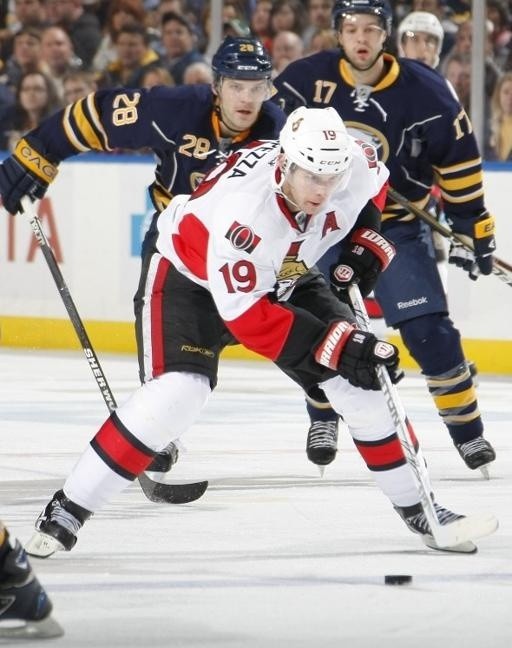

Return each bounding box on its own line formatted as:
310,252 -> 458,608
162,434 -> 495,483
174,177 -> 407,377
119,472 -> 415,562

306,407 -> 344,479
22,489 -> 93,559
0,537 -> 65,638
392,492 -> 469,542
143,438 -> 180,486
446,423 -> 496,481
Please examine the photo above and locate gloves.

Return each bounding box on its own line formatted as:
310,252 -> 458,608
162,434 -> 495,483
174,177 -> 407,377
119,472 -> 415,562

0,129 -> 64,216
328,225 -> 398,305
444,212 -> 498,280
312,319 -> 405,392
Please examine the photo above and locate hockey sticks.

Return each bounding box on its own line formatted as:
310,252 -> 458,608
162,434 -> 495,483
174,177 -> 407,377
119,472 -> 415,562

18,193 -> 208,505
347,280 -> 500,549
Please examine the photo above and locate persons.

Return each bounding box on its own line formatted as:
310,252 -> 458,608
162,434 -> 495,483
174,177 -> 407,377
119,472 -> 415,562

266,0 -> 495,471
1,34 -> 342,466
1,1 -> 512,164
23,108 -> 496,558
1,520 -> 52,625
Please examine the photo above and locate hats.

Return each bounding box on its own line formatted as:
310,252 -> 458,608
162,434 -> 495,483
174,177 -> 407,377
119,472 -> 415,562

160,11 -> 196,32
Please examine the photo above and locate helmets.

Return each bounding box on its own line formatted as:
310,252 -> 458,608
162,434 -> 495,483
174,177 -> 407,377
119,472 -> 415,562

395,10 -> 445,70
278,105 -> 354,193
329,0 -> 393,38
210,33 -> 273,102
